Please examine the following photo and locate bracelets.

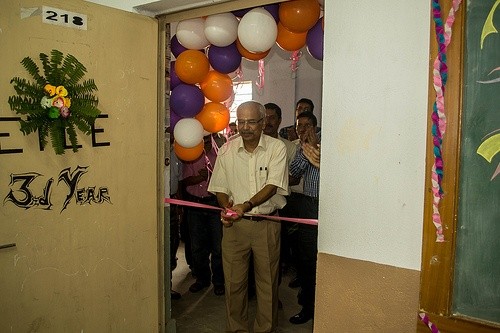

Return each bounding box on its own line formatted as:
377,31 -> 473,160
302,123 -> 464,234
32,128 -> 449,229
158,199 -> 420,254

243,201 -> 253,212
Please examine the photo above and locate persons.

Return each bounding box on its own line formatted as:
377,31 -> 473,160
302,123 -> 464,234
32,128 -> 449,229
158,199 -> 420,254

210,102 -> 287,333
169,96 -> 323,328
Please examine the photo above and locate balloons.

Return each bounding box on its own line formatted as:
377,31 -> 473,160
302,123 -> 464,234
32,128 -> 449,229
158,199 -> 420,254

195,103 -> 230,133
173,119 -> 205,148
166,2 -> 278,116
168,111 -> 183,135
274,20 -> 307,51
307,19 -> 324,62
172,138 -> 205,162
277,1 -> 321,33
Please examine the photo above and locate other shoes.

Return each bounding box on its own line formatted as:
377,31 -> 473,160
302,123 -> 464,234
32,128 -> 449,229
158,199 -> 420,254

189,280 -> 211,292
213,282 -> 225,295
288,277 -> 300,288
171,290 -> 181,300
277,299 -> 283,309
289,308 -> 314,324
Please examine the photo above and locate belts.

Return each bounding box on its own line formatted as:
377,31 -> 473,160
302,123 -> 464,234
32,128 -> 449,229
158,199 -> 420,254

243,210 -> 278,221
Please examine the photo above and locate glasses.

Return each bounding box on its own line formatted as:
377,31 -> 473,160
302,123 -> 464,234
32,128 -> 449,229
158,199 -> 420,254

235,118 -> 263,125
296,107 -> 311,114
